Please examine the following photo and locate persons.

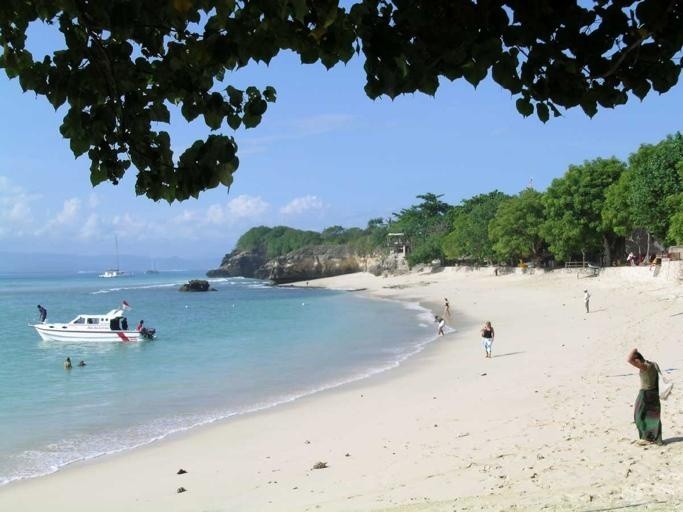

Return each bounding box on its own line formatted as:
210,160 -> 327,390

443,297 -> 449,315
433,316 -> 445,337
627,346 -> 663,448
36,304 -> 47,322
137,320 -> 147,335
121,317 -> 128,330
583,289 -> 590,315
63,356 -> 71,369
78,361 -> 86,367
479,321 -> 494,357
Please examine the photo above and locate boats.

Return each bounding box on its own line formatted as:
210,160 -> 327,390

28,302 -> 157,343
100,269 -> 129,278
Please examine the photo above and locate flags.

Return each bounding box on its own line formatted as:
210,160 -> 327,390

122,299 -> 132,313
